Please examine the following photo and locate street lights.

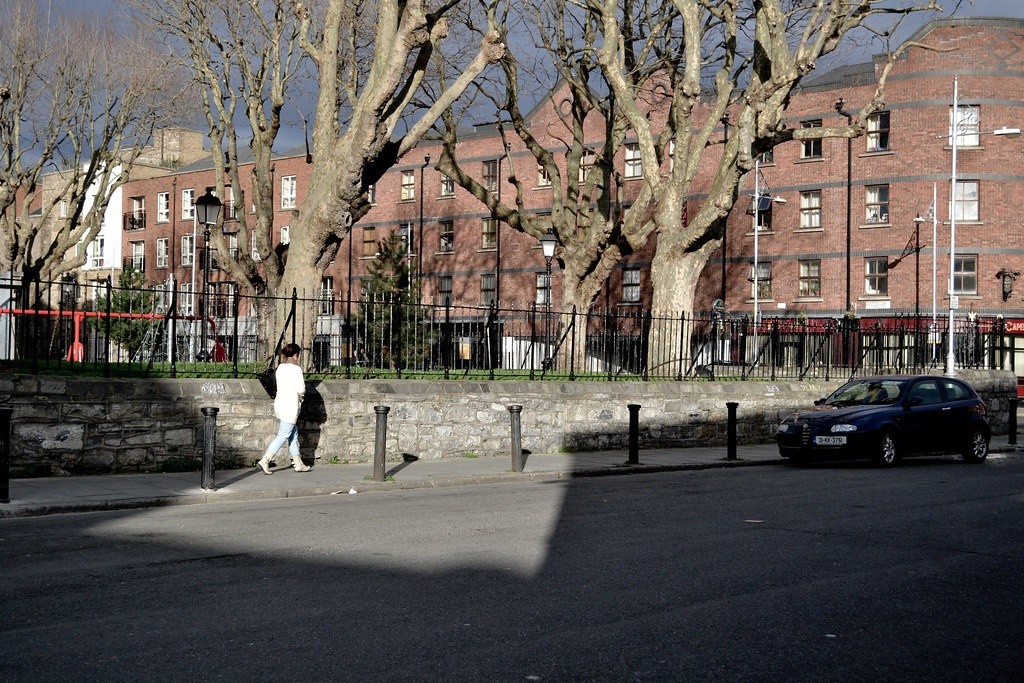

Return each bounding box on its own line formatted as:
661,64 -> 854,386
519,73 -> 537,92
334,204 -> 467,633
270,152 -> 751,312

887,213 -> 926,328
60,271 -> 75,361
935,77 -> 1021,389
191,187 -> 224,362
538,227 -> 558,372
748,161 -> 787,369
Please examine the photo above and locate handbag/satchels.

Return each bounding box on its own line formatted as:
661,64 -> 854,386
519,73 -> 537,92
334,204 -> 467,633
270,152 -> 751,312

258,367 -> 278,399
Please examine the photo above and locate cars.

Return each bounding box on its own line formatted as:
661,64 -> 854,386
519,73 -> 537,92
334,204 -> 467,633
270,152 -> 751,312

776,374 -> 992,468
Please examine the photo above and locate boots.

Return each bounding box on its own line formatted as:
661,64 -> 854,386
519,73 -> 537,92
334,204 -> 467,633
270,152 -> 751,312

257,450 -> 275,473
292,455 -> 310,471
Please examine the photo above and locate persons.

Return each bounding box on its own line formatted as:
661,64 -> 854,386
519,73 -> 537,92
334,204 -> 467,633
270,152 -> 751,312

256,343 -> 311,475
